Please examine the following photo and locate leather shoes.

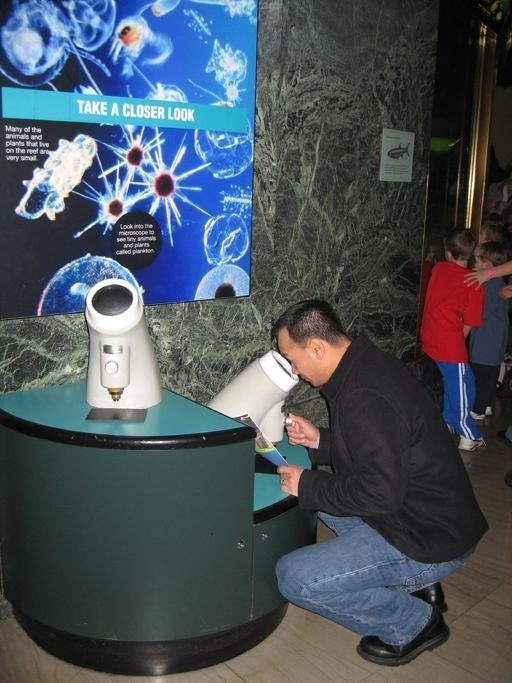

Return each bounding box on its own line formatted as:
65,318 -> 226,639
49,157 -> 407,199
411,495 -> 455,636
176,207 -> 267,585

413,581 -> 452,617
356,609 -> 448,668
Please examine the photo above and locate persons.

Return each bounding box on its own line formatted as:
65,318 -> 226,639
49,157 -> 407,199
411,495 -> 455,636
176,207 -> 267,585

267,297 -> 491,667
461,186 -> 512,488
419,226 -> 487,452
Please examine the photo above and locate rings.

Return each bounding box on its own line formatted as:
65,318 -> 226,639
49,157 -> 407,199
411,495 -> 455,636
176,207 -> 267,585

281,479 -> 286,485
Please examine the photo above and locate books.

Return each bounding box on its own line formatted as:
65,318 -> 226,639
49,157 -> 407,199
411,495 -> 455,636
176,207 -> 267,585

232,413 -> 289,468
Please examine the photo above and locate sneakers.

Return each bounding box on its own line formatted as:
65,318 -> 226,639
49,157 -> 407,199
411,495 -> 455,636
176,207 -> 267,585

444,401 -> 511,451
